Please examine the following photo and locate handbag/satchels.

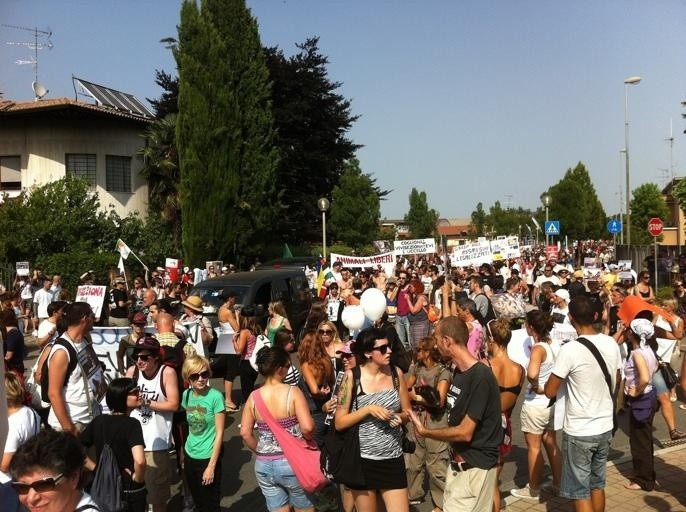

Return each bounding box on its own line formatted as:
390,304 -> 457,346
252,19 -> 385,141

323,417 -> 367,490
616,405 -> 635,438
282,430 -> 332,495
214,325 -> 224,340
170,410 -> 189,449
658,355 -> 677,388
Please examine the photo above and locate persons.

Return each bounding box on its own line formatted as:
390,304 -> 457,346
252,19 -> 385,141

0,233 -> 685,510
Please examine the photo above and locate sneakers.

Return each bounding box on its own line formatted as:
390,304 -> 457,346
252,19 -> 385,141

182,495 -> 196,512
511,483 -> 541,501
543,483 -> 561,494
31,330 -> 39,336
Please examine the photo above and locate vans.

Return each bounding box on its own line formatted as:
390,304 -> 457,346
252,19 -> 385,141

179,268 -> 312,346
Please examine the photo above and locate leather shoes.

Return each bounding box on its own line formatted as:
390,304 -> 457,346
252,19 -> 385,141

670,391 -> 677,402
679,404 -> 686,409
669,428 -> 686,439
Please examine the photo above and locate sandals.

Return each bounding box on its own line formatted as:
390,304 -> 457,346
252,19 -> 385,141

225,403 -> 240,413
23,328 -> 29,334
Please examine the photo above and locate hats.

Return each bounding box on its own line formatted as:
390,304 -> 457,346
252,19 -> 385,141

351,255 -> 620,321
181,296 -> 204,313
241,305 -> 257,316
174,324 -> 190,340
114,276 -> 127,283
128,335 -> 160,355
183,263 -> 235,274
129,311 -> 147,324
156,266 -> 165,271
335,339 -> 358,356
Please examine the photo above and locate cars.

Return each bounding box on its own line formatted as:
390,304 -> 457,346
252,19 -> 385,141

254,256 -> 322,274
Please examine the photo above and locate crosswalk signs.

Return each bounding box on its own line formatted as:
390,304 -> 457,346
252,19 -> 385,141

544,221 -> 560,236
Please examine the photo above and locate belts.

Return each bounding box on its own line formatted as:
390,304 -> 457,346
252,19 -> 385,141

448,453 -> 476,472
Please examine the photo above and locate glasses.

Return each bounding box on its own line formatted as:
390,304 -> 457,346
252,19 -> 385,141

127,386 -> 140,396
10,471 -> 68,495
187,370 -> 210,380
318,329 -> 336,335
368,343 -> 392,355
132,352 -> 156,362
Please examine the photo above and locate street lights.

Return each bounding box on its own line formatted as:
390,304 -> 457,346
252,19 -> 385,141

624,76 -> 644,247
316,197 -> 331,264
540,191 -> 554,245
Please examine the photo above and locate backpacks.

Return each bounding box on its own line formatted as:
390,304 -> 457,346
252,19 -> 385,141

91,439 -> 127,511
249,333 -> 273,373
156,338 -> 187,383
40,338 -> 77,403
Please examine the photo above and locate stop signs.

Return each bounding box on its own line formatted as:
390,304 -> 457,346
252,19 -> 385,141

648,218 -> 664,237
